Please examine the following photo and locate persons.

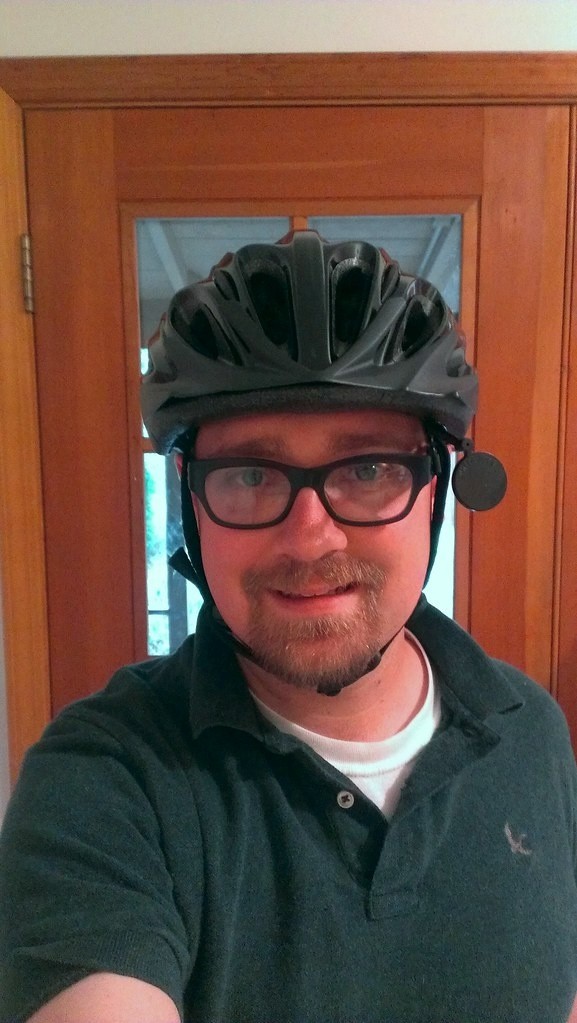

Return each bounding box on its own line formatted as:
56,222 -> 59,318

0,229 -> 577,1023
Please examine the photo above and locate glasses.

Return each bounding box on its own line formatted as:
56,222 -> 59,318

180,448 -> 434,530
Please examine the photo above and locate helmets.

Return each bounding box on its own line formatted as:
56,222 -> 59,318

139,229 -> 479,455
